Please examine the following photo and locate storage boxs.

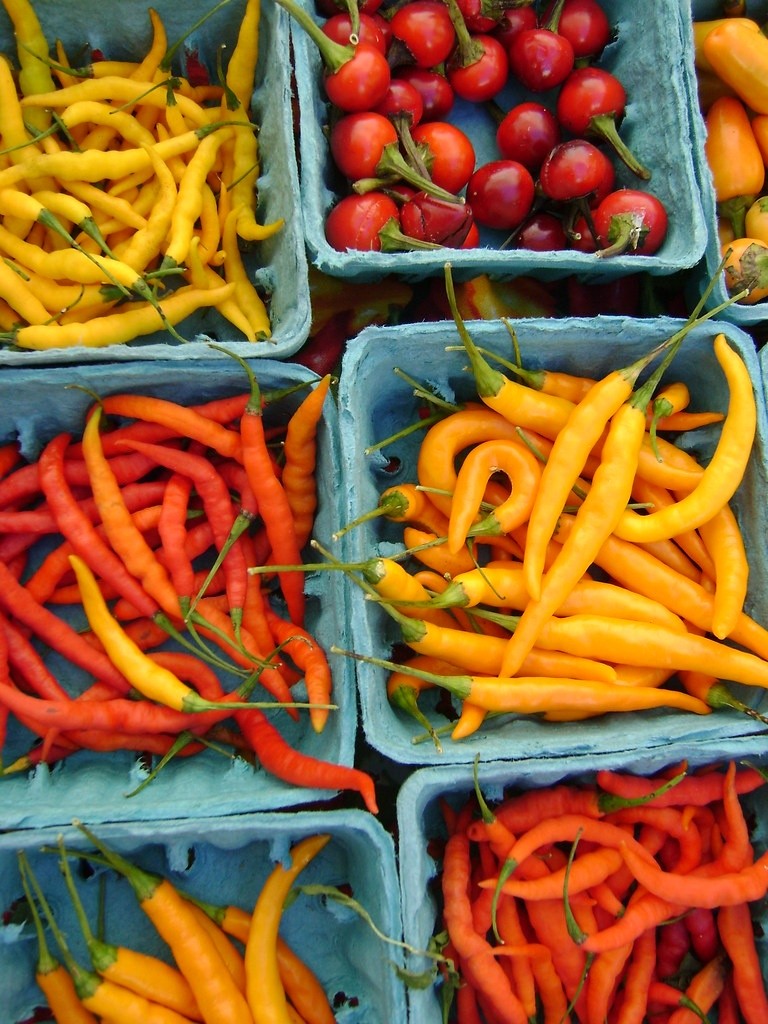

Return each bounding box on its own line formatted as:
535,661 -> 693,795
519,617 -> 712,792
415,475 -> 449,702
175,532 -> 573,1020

0,0 -> 768,1024
